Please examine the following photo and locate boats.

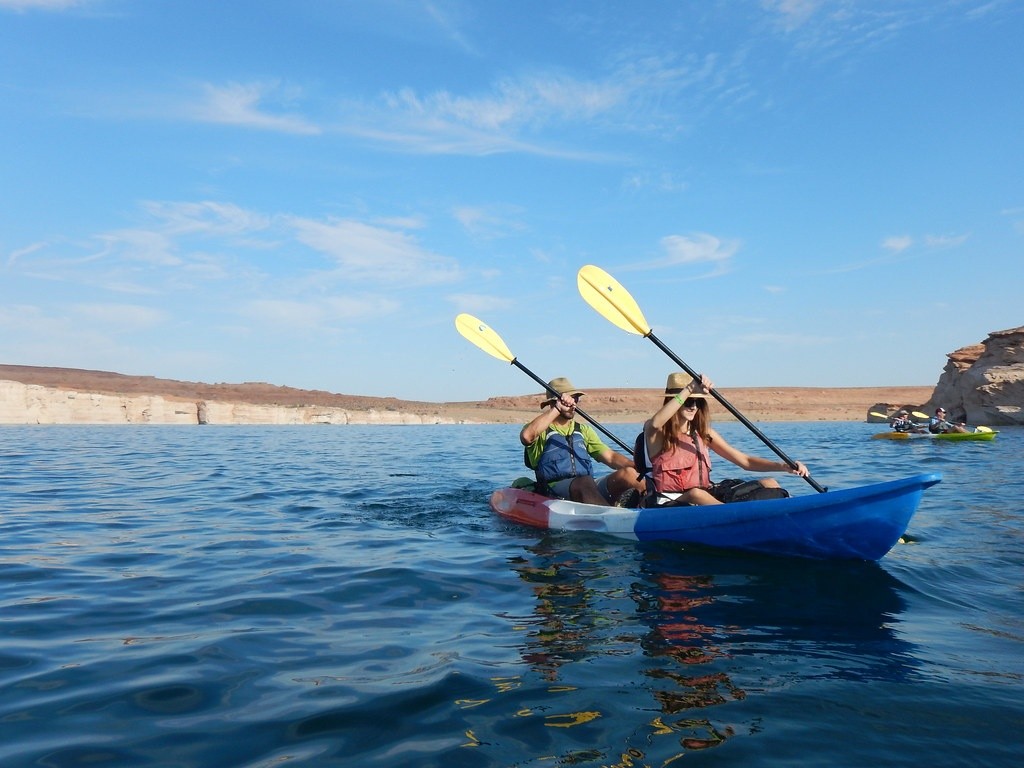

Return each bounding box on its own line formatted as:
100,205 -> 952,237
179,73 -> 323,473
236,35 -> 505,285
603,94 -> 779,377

489,472 -> 942,563
872,429 -> 1000,441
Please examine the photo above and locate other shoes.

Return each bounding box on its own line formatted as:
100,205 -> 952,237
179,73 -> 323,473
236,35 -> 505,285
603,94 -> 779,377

620,488 -> 639,508
973,428 -> 978,433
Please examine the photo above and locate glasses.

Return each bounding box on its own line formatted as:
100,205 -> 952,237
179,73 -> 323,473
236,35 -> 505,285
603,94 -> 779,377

572,396 -> 580,404
681,399 -> 703,408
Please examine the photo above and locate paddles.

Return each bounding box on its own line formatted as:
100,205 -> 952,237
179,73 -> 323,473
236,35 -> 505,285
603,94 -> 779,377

454,311 -> 636,456
910,410 -> 993,433
869,411 -> 929,428
576,262 -> 833,494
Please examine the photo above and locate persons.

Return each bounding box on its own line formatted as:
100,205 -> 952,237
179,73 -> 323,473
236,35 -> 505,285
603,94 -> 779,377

520,378 -> 647,509
890,410 -> 929,434
643,372 -> 809,507
929,407 -> 966,434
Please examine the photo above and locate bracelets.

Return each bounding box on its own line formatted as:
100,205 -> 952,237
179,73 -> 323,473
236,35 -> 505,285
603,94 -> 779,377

555,405 -> 562,413
676,395 -> 684,404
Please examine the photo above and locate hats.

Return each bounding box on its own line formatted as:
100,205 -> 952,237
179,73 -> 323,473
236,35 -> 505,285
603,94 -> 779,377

541,378 -> 588,410
936,407 -> 947,414
658,373 -> 714,399
899,410 -> 910,415
